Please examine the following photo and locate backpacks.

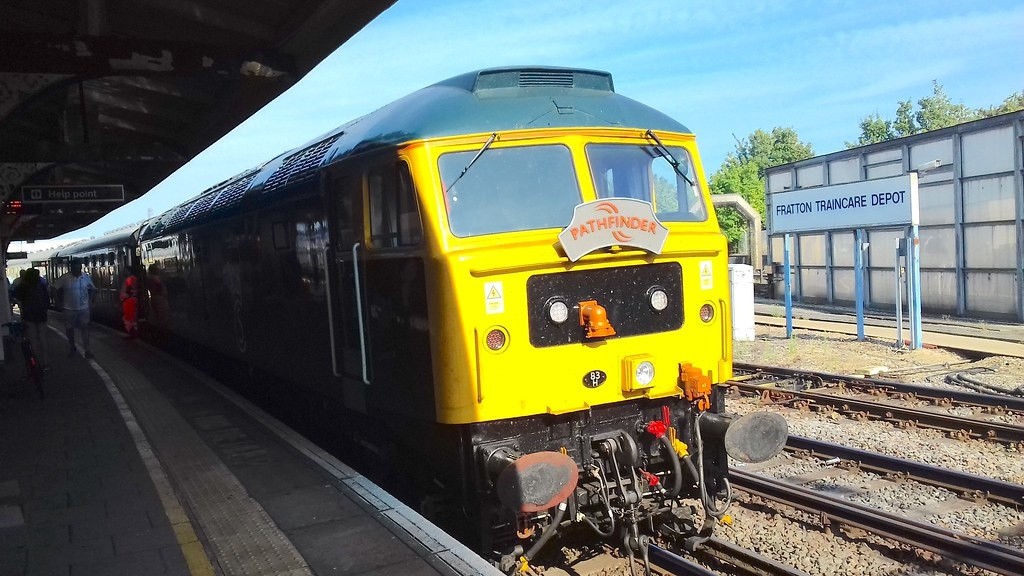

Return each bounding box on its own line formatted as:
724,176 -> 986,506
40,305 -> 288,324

19,275 -> 49,324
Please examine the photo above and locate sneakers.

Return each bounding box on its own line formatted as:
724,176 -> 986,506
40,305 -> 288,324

69,346 -> 76,351
84,350 -> 96,359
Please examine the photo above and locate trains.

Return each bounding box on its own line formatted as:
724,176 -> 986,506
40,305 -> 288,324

31,64 -> 792,576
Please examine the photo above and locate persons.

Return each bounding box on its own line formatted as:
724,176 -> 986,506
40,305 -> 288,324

51,261 -> 97,359
13,269 -> 52,375
148,264 -> 170,324
121,266 -> 141,340
